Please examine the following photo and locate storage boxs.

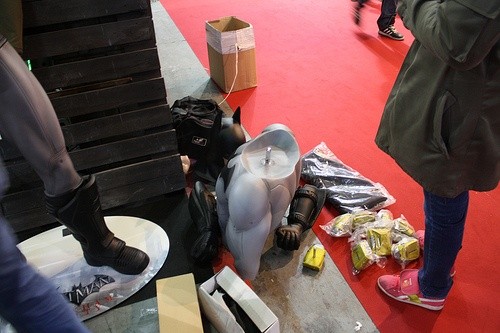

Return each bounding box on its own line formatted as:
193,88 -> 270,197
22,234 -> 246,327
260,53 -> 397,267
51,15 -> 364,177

205,15 -> 258,93
156,266 -> 280,333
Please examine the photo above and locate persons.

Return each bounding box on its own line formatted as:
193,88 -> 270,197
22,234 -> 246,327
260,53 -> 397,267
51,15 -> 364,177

0,33 -> 149,275
374,0 -> 500,310
0,216 -> 90,333
350,0 -> 404,40
184,106 -> 325,279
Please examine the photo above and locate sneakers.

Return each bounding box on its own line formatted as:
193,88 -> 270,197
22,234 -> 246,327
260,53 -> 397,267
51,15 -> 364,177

378,24 -> 404,40
416,230 -> 456,277
352,2 -> 365,24
377,269 -> 447,310
